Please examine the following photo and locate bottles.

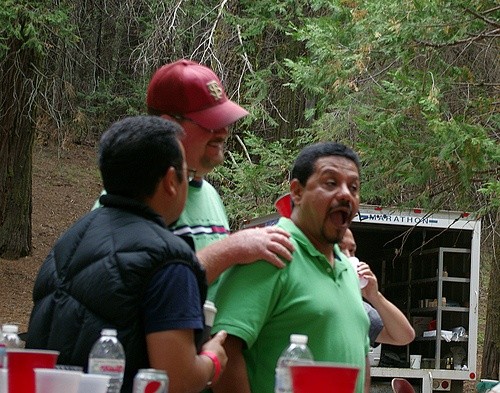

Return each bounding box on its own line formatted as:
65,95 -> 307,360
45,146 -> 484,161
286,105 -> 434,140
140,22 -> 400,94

0,324 -> 25,369
274,334 -> 314,393
89,328 -> 125,393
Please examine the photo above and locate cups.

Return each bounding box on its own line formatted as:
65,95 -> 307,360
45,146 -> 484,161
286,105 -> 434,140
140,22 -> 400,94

79,374 -> 110,393
348,257 -> 368,288
410,355 -> 421,369
197,300 -> 217,355
32,367 -> 81,393
5,349 -> 61,393
287,365 -> 360,393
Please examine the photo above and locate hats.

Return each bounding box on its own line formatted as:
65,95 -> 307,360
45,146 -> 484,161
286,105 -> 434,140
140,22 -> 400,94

146,60 -> 249,132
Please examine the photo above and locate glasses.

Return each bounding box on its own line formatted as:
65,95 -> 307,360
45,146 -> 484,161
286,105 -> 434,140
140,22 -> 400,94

181,166 -> 198,184
173,112 -> 224,136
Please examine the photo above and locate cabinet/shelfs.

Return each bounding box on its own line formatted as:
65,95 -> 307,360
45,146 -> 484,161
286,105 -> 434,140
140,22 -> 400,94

381,246 -> 472,369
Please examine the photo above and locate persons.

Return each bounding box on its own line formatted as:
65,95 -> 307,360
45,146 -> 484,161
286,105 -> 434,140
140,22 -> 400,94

90,60 -> 294,304
338,229 -> 415,348
26,116 -> 228,393
211,145 -> 370,393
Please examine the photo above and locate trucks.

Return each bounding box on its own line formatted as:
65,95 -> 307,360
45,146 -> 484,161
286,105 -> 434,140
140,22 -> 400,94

238,204 -> 482,392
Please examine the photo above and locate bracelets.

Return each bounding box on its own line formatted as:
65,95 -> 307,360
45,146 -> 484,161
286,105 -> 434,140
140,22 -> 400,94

201,351 -> 220,387
